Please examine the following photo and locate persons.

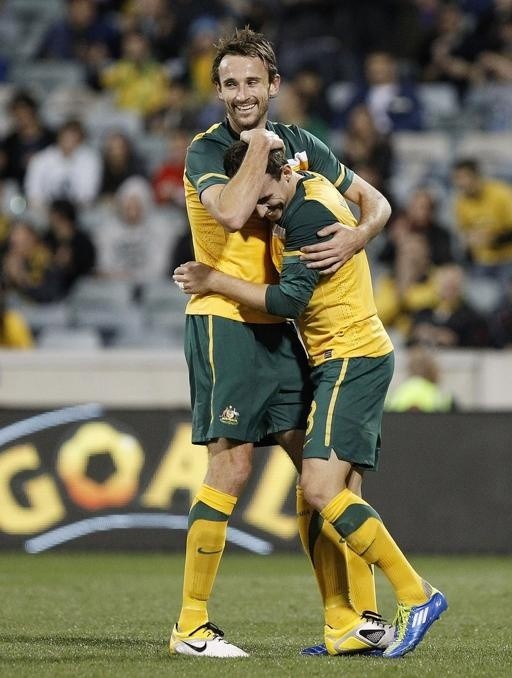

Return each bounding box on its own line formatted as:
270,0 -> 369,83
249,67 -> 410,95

161,21 -> 392,661
168,130 -> 450,662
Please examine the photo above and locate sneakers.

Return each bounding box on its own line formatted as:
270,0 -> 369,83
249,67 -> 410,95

169,622 -> 250,659
323,610 -> 396,656
381,586 -> 448,659
